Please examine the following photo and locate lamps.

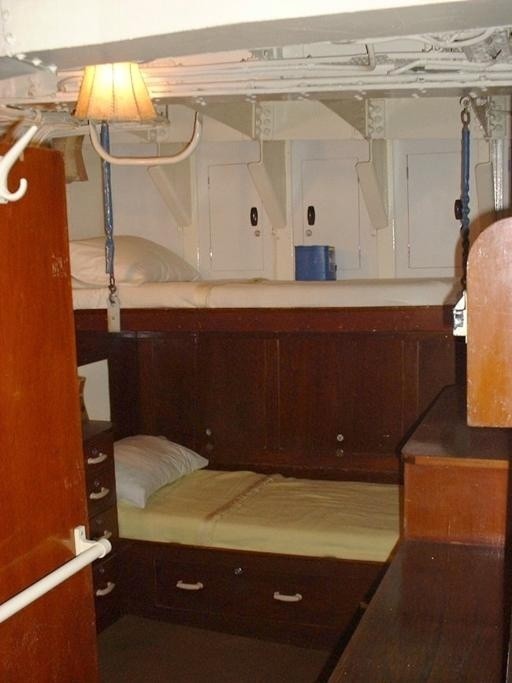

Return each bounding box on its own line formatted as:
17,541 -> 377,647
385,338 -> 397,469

72,60 -> 159,123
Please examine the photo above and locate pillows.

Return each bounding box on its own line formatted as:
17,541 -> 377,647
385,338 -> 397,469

66,234 -> 202,284
112,433 -> 209,510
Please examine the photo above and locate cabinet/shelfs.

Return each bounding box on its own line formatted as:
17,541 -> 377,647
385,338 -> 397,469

116,537 -> 390,644
133,331 -> 468,470
189,134 -> 392,278
327,211 -> 511,683
385,131 -> 483,279
81,419 -> 124,632
1,141 -> 110,681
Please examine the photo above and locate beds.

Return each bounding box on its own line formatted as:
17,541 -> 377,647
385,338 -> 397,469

60,230 -> 465,334
116,467 -> 402,645
